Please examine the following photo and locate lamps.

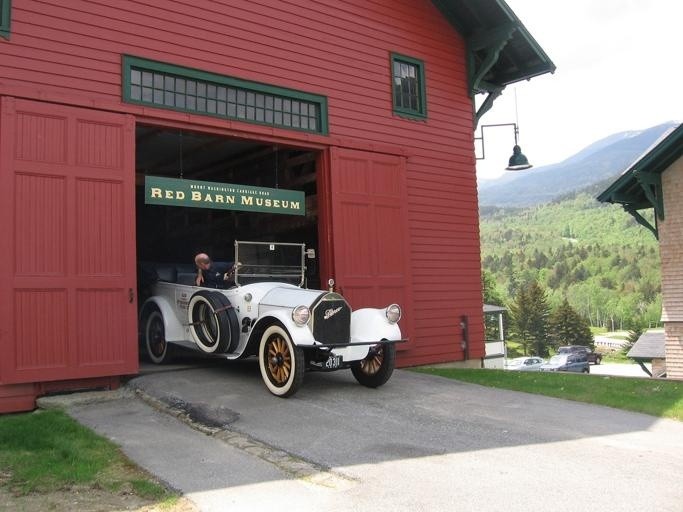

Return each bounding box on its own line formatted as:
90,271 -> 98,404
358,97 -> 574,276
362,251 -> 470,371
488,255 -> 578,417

470,123 -> 534,171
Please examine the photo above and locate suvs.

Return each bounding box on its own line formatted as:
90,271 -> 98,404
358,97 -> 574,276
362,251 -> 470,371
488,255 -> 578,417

507,354 -> 590,374
559,345 -> 602,365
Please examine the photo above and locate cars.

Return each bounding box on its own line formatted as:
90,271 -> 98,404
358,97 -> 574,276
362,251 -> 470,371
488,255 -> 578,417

139,239 -> 410,399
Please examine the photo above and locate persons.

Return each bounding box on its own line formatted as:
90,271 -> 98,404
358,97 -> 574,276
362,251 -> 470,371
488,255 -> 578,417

195,253 -> 244,289
